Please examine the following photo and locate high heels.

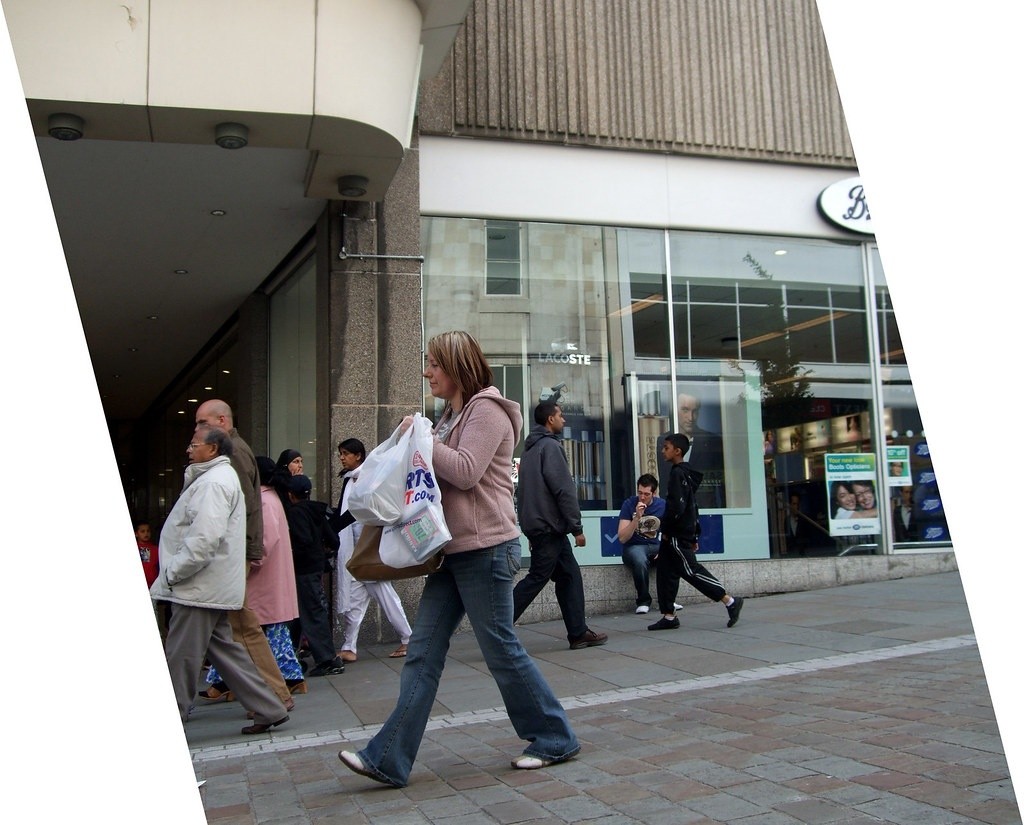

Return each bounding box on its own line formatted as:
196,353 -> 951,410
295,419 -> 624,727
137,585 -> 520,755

289,680 -> 307,694
199,681 -> 234,702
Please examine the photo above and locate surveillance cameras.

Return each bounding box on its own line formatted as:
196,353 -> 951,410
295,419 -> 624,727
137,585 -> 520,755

551,382 -> 566,392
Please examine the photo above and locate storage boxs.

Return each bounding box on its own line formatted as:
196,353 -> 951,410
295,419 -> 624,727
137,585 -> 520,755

774,452 -> 800,481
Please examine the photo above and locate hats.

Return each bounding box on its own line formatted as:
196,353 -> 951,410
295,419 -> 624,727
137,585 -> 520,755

286,475 -> 312,493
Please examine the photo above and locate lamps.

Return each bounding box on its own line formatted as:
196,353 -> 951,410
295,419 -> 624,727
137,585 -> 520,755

338,176 -> 369,197
48,114 -> 83,141
722,337 -> 738,349
215,124 -> 248,149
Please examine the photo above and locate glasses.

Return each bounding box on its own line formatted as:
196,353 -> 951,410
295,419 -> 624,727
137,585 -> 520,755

188,442 -> 211,450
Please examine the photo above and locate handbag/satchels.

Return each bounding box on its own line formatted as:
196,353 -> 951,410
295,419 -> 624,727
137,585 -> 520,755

343,413 -> 454,582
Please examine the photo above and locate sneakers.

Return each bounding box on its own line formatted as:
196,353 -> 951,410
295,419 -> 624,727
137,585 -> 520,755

309,657 -> 345,675
726,597 -> 744,629
569,630 -> 609,649
647,616 -> 680,631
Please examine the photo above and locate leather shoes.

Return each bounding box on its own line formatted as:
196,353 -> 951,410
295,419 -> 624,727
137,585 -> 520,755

242,715 -> 291,734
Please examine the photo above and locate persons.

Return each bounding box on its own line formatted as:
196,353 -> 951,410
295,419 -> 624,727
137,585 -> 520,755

648,433 -> 743,631
656,385 -> 726,508
830,481 -> 878,520
619,473 -> 683,614
137,400 -> 416,735
514,402 -> 608,650
894,487 -> 919,541
338,330 -> 581,788
785,494 -> 810,550
847,415 -> 861,441
890,462 -> 903,476
765,429 -> 775,454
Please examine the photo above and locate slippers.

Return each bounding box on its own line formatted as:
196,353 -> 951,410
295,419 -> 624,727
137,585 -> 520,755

389,645 -> 408,658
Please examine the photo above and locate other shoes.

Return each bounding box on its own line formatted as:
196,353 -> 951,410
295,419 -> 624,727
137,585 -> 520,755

338,749 -> 408,787
247,705 -> 295,719
636,606 -> 650,613
674,603 -> 683,610
510,743 -> 582,770
